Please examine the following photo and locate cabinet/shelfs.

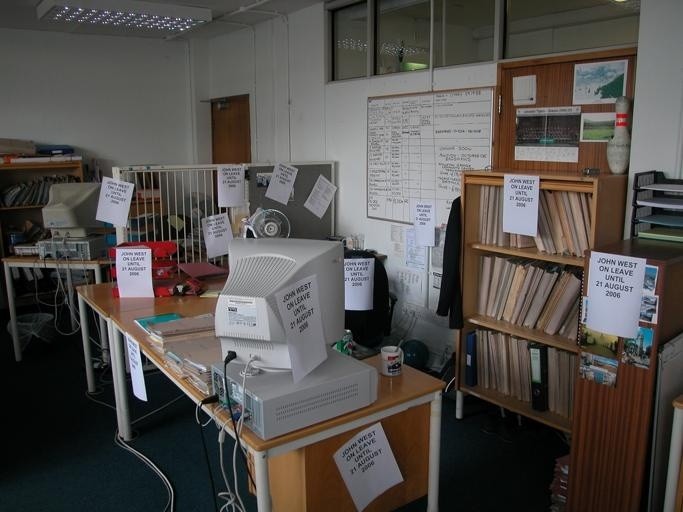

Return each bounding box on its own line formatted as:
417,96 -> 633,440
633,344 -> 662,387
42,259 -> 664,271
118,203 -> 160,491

0,162 -> 93,311
454,168 -> 626,434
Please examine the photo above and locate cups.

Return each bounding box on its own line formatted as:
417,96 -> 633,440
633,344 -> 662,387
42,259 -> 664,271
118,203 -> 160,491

380,346 -> 404,377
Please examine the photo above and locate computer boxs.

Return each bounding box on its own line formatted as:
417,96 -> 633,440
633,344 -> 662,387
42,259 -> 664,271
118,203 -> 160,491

211,350 -> 379,441
38,240 -> 107,261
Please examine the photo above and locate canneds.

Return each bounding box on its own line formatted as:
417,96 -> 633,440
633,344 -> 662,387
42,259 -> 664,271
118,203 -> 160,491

335,329 -> 353,357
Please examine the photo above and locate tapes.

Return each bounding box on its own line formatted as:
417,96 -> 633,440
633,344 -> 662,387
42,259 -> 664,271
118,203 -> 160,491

402,63 -> 427,71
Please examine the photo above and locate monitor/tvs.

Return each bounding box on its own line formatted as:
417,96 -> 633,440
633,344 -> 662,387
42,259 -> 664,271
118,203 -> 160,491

41,182 -> 109,240
214,236 -> 348,374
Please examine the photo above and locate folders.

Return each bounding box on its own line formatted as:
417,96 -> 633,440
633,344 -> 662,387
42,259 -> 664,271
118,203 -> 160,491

528,343 -> 547,412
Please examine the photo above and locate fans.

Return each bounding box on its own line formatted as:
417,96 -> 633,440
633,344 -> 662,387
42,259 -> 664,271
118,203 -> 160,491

251,209 -> 290,238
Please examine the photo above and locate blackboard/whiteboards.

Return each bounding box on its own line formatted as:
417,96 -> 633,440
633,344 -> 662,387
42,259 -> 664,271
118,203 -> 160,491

368,87 -> 493,228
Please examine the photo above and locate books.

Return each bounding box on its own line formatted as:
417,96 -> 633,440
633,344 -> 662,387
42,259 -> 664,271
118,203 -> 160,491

461,185 -> 592,422
132,311 -> 222,397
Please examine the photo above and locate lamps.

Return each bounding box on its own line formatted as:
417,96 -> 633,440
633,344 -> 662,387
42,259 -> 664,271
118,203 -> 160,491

35,0 -> 212,34
